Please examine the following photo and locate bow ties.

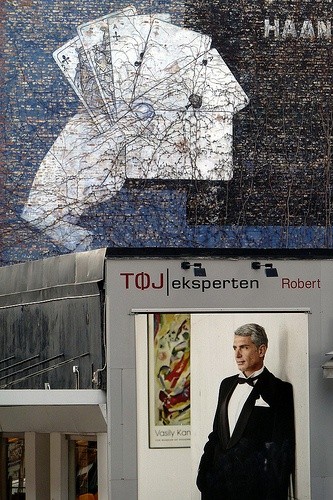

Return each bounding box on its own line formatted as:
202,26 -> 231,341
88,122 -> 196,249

236,376 -> 258,388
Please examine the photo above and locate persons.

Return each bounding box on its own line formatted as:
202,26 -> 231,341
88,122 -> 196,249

196,322 -> 296,500
